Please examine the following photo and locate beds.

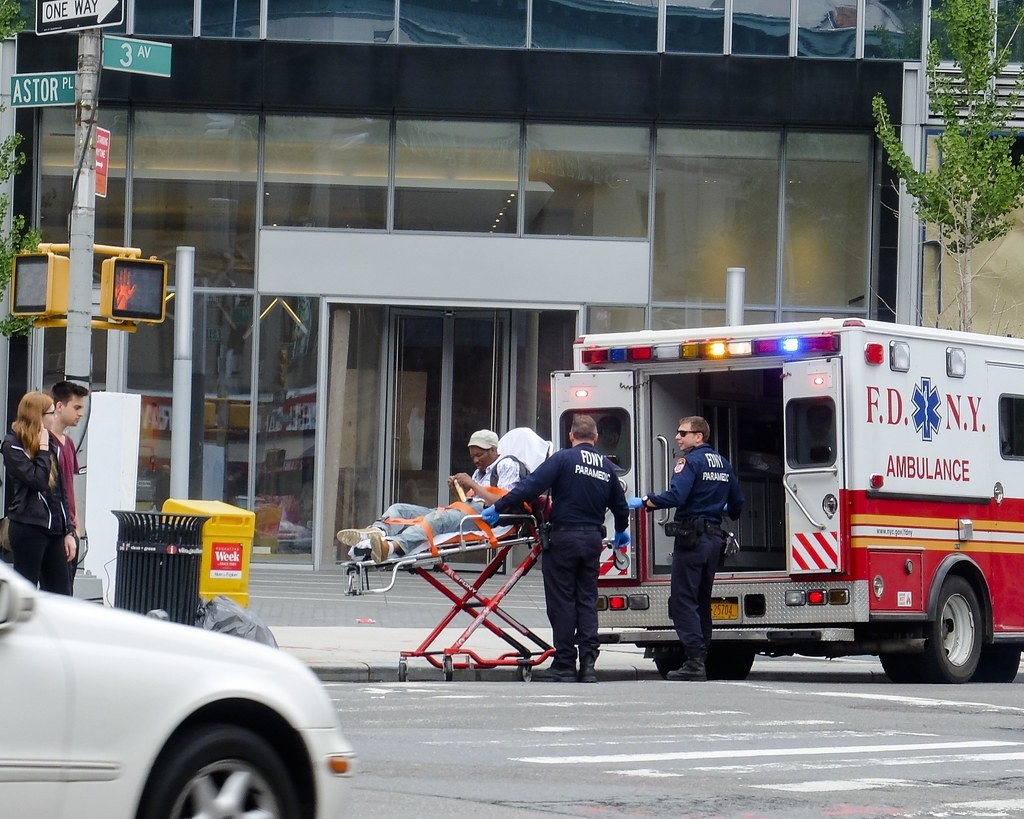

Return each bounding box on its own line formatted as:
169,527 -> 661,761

342,428 -> 631,682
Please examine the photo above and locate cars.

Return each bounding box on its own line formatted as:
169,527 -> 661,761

0,563 -> 358,819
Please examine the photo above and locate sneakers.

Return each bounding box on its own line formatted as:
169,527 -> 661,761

578,654 -> 597,682
667,657 -> 707,680
532,656 -> 577,682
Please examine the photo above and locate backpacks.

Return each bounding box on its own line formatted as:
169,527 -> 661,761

490,455 -> 538,522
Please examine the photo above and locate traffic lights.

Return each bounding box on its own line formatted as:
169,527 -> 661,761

101,257 -> 167,325
11,251 -> 70,318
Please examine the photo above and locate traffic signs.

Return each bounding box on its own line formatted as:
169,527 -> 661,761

36,0 -> 125,37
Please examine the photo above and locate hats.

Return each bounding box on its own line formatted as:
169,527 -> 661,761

468,430 -> 498,449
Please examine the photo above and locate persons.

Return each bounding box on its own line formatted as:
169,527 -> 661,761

226,466 -> 243,506
337,429 -> 531,564
2,381 -> 89,597
481,414 -> 630,682
628,415 -> 746,681
152,465 -> 169,511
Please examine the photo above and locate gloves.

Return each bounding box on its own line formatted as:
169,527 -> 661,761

614,530 -> 629,548
482,506 -> 499,525
627,498 -> 643,508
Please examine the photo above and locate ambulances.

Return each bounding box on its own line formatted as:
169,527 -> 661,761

550,316 -> 1024,682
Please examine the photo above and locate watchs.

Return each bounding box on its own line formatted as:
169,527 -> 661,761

69,532 -> 77,538
642,495 -> 649,507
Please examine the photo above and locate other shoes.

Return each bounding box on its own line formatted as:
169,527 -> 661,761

337,525 -> 388,546
371,533 -> 389,563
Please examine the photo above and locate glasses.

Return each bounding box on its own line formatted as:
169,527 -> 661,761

43,410 -> 55,414
677,429 -> 704,440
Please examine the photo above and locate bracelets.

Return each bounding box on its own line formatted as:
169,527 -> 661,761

39,443 -> 49,446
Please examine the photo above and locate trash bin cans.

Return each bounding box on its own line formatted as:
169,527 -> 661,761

109,510 -> 212,627
